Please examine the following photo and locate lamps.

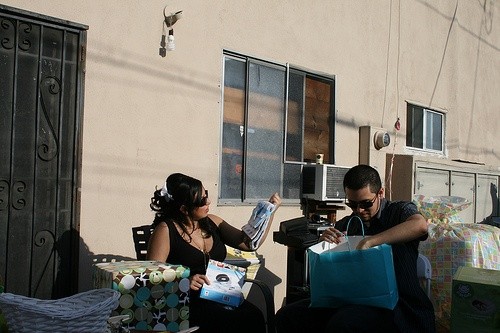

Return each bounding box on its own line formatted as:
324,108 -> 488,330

163,5 -> 183,51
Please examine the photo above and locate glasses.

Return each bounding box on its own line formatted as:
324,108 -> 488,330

193,190 -> 209,207
344,192 -> 378,210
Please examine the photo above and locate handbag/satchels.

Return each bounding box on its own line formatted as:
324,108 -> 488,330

308,216 -> 399,310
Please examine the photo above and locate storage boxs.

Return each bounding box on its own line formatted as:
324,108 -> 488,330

94,261 -> 191,333
451,266 -> 500,333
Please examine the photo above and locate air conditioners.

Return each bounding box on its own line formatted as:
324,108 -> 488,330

303,163 -> 353,202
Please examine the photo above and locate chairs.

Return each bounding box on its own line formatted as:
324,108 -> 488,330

132,225 -> 274,332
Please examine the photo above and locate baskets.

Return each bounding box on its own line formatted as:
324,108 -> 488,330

0,288 -> 121,333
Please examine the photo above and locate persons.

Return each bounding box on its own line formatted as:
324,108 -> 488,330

145,173 -> 282,333
277,165 -> 435,333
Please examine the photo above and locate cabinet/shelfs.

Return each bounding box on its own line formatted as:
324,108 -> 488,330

386,152 -> 500,223
